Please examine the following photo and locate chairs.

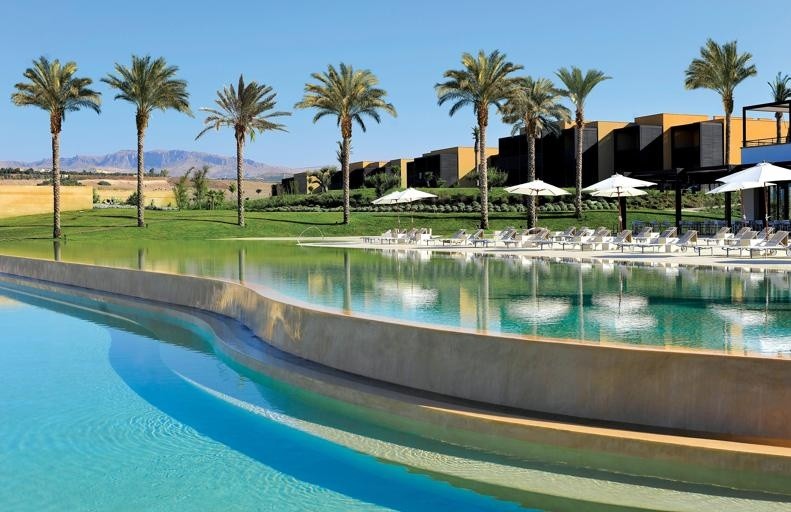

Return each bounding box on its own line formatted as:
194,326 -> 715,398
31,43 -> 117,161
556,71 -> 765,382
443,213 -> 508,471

361,218 -> 791,259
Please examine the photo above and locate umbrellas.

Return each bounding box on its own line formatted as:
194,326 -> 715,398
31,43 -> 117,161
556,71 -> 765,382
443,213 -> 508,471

398,187 -> 439,222
705,179 -> 778,224
505,177 -> 573,232
591,188 -> 649,197
372,190 -> 408,224
715,159 -> 791,236
581,171 -> 659,228
